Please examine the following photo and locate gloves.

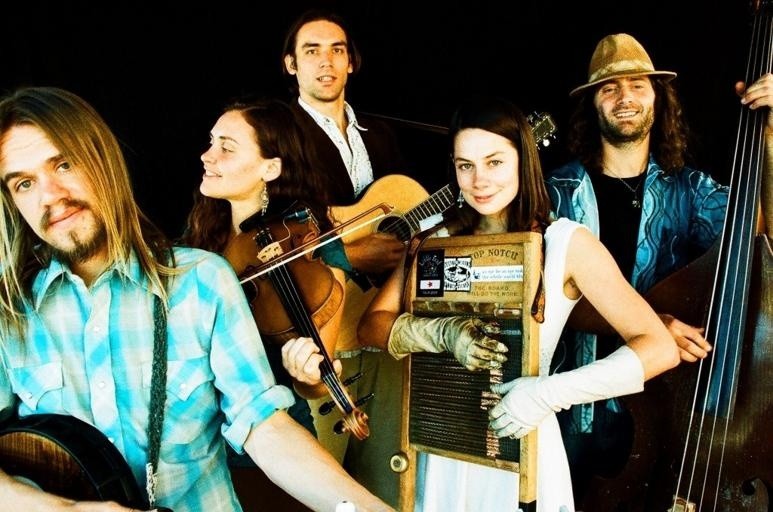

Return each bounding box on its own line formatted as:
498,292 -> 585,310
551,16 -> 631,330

384,310 -> 511,374
484,342 -> 647,441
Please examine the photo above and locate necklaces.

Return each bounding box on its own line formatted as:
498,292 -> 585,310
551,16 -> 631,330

600,162 -> 647,208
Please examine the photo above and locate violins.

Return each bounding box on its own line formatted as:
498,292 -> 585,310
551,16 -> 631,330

225,204 -> 374,442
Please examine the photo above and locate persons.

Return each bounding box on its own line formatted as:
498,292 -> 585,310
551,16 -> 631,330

542,33 -> 773,512
0,85 -> 399,512
356,95 -> 680,512
176,95 -> 354,512
277,7 -> 420,469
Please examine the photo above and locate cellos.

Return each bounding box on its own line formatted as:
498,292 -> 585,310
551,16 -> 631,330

618,0 -> 773,512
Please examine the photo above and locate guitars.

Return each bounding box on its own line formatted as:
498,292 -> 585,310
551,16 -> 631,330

325,110 -> 560,246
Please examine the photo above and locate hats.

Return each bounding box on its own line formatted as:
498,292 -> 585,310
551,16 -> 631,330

565,28 -> 680,103
